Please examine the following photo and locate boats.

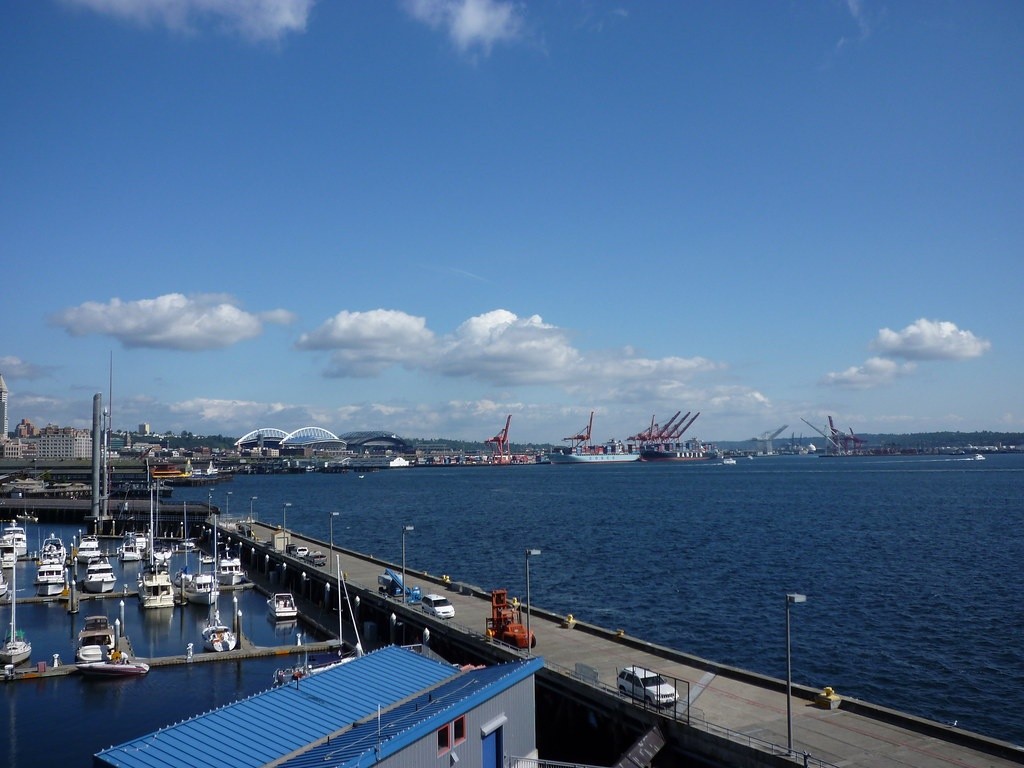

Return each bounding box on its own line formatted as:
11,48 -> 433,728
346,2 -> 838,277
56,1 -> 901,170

548,438 -> 736,464
975,454 -> 986,461
76,615 -> 150,675
0,510 -> 148,597
153,542 -> 298,619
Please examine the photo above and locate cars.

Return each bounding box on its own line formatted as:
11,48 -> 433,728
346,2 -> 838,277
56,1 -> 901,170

330,512 -> 340,573
291,546 -> 328,566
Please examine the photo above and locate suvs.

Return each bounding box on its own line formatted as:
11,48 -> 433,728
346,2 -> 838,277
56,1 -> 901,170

422,594 -> 455,619
616,666 -> 680,708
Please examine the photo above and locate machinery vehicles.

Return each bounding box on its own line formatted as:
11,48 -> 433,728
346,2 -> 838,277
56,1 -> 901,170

378,568 -> 423,605
486,589 -> 536,648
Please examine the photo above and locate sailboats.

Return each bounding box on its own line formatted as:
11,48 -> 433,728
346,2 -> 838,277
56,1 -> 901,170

137,487 -> 176,609
173,501 -> 194,588
0,537 -> 31,665
273,555 -> 364,685
201,514 -> 237,652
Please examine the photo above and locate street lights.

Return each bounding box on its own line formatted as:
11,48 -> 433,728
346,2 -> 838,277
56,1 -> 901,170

208,488 -> 292,554
403,526 -> 414,603
786,594 -> 807,754
528,550 -> 541,653
